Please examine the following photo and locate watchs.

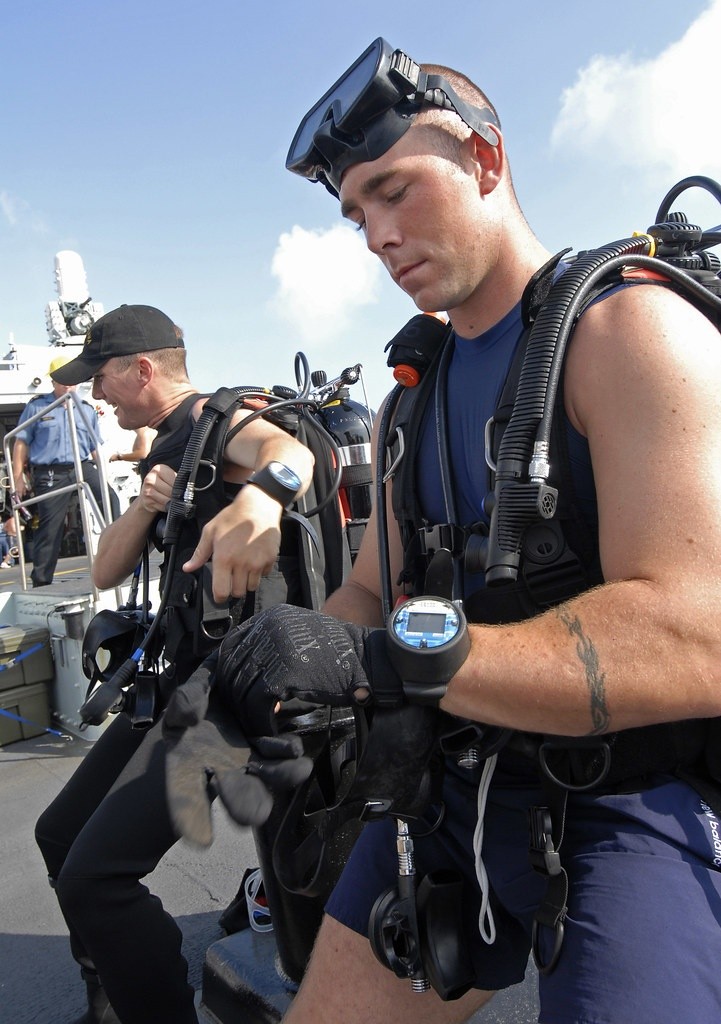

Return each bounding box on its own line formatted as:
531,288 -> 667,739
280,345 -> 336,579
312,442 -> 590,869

387,597 -> 472,711
245,460 -> 323,559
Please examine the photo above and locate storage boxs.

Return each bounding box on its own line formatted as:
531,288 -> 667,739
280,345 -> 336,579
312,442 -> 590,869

0,683 -> 51,747
1,623 -> 55,691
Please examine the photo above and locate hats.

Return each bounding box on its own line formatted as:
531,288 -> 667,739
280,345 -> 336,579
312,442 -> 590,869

47,357 -> 70,376
50,303 -> 185,386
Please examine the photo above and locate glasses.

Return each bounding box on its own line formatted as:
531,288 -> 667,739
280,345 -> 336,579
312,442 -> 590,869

285,36 -> 499,202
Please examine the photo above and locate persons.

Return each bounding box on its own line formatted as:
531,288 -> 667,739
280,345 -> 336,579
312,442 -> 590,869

34,305 -> 315,1024
162,64 -> 721,1024
14,357 -> 120,589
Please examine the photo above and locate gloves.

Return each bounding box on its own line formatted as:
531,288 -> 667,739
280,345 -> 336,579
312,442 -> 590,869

161,646 -> 313,848
216,603 -> 406,729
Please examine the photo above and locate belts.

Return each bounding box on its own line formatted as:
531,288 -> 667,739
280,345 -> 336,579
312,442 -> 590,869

35,458 -> 89,473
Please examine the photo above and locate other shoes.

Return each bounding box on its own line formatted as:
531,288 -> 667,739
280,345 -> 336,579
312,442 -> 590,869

0,562 -> 11,568
74,969 -> 122,1024
